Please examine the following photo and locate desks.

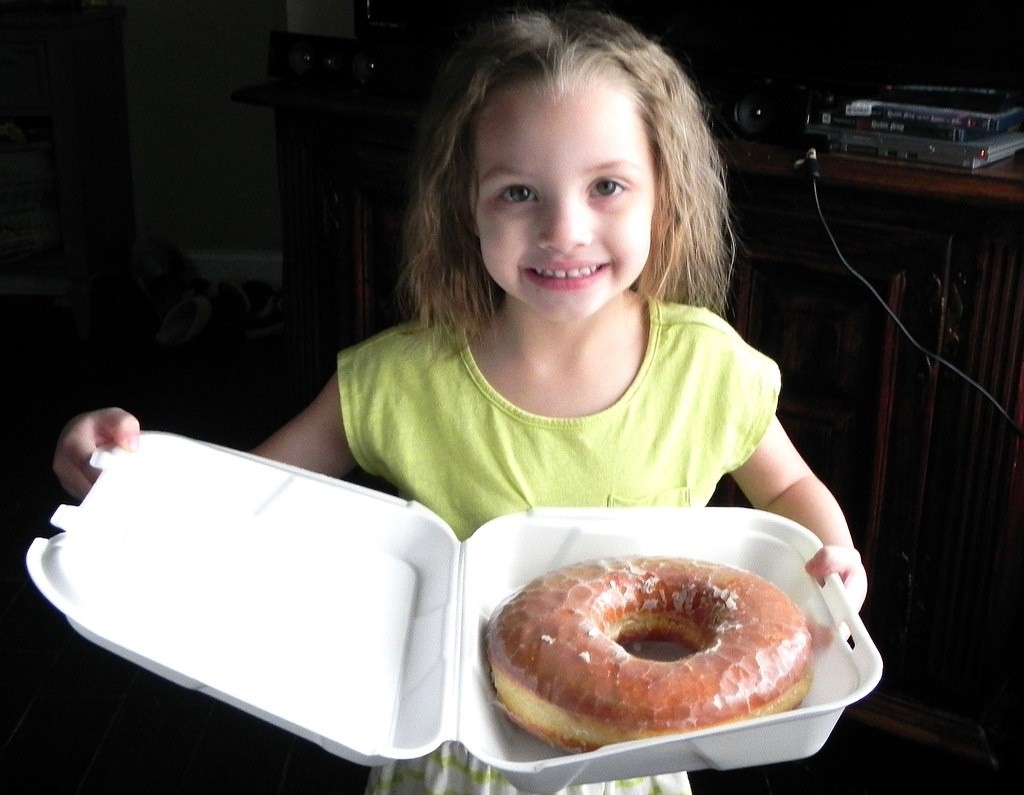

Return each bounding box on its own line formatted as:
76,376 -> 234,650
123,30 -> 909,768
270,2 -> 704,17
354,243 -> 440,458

232,41 -> 1023,776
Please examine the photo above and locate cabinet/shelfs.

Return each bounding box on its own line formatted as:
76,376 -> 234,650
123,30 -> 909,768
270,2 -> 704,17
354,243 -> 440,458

0,6 -> 135,341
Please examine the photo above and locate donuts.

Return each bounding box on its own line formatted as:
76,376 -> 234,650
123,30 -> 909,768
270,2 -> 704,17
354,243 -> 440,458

485,554 -> 814,756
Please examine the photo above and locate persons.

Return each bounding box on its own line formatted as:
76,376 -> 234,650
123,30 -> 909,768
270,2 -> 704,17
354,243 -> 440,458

52,1 -> 867,795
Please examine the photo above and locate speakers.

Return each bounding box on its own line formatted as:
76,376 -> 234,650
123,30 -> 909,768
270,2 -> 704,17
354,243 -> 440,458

267,29 -> 391,96
730,77 -> 837,151
